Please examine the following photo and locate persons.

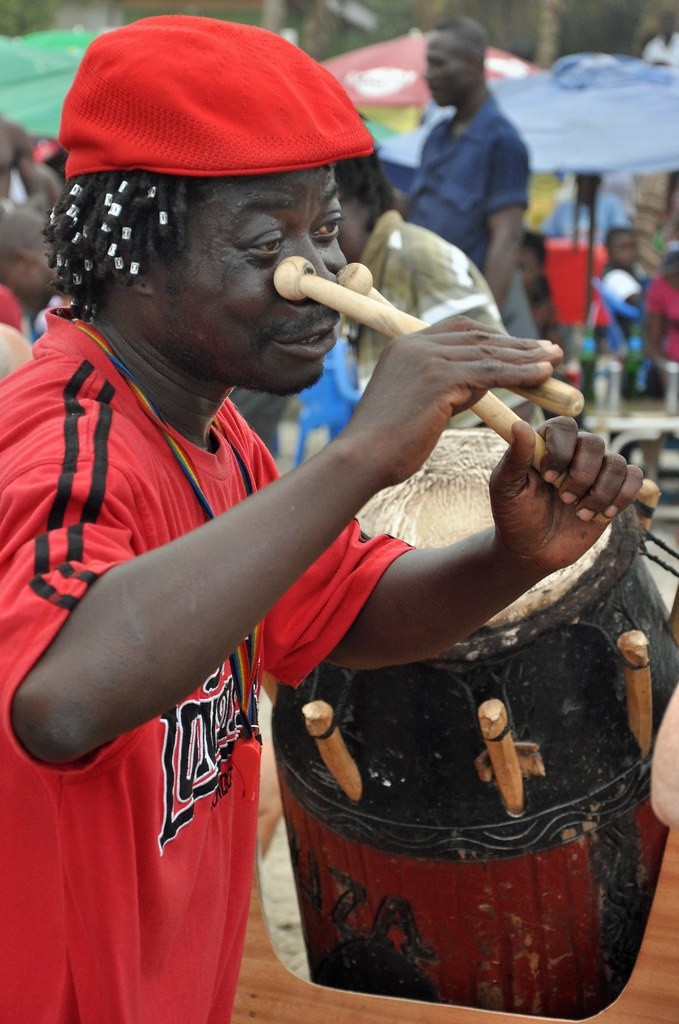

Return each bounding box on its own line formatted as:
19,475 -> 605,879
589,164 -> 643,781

0,117 -> 65,384
640,251 -> 679,417
330,109 -> 545,441
538,172 -> 634,242
587,227 -> 652,414
0,15 -> 644,1024
390,14 -> 533,307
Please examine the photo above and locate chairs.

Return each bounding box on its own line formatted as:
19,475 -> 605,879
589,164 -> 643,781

290,274 -> 679,465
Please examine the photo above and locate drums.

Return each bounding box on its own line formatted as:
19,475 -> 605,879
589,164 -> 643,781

270,420 -> 679,1023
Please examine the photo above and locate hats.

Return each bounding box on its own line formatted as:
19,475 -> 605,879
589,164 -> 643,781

58,11 -> 374,181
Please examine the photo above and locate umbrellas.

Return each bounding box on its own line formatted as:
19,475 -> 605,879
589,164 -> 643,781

316,28 -> 679,323
